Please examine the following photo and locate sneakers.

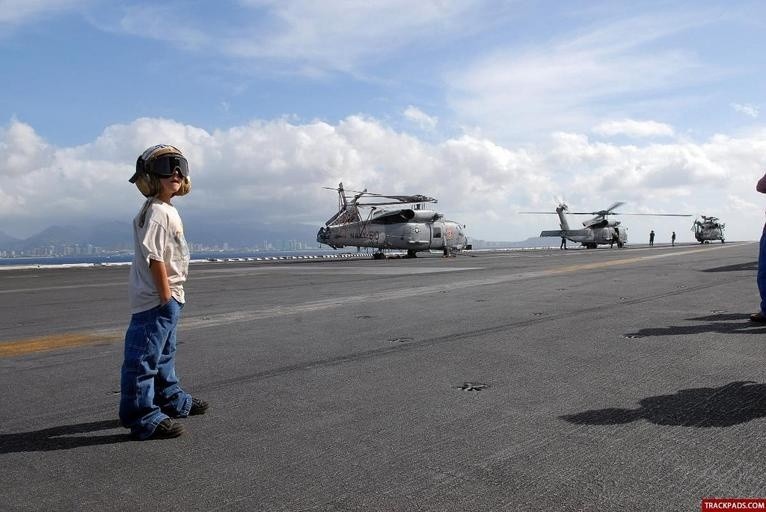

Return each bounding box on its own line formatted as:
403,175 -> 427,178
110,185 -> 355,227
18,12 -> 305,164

162,390 -> 208,416
132,414 -> 185,440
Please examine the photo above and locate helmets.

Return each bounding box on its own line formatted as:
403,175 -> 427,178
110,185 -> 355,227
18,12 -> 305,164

132,143 -> 193,198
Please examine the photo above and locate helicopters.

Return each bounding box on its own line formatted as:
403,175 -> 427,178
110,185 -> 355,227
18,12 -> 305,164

689,213 -> 728,244
515,193 -> 693,249
315,180 -> 472,259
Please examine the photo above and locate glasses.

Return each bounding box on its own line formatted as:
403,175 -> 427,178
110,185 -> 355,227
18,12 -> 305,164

152,155 -> 190,178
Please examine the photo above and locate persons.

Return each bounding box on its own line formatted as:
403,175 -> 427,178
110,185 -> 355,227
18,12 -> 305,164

751,177 -> 766,323
119,144 -> 209,439
671,232 -> 674,244
650,229 -> 656,244
561,237 -> 567,250
